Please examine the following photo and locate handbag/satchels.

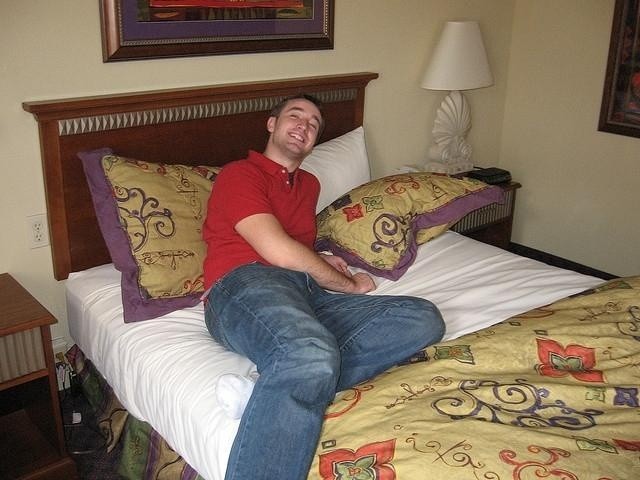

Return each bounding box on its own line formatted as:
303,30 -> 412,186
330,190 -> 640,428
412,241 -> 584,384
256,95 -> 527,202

55,361 -> 87,407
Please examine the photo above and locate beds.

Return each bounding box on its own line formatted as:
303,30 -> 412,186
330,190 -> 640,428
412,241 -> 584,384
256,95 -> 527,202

67,229 -> 638,479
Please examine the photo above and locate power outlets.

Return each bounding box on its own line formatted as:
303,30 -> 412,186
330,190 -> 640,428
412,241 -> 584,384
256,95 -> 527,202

24,213 -> 49,251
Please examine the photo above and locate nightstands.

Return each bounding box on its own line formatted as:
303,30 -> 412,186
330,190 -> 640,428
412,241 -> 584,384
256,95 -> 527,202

451,168 -> 523,249
0,270 -> 78,479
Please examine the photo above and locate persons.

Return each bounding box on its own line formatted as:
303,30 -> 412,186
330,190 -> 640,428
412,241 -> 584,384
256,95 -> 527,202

204,93 -> 444,479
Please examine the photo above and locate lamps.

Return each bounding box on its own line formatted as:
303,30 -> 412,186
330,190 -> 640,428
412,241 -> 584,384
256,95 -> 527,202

419,16 -> 497,177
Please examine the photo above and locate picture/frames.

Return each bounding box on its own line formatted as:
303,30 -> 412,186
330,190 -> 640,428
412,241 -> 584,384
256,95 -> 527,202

96,0 -> 337,65
594,1 -> 639,139
